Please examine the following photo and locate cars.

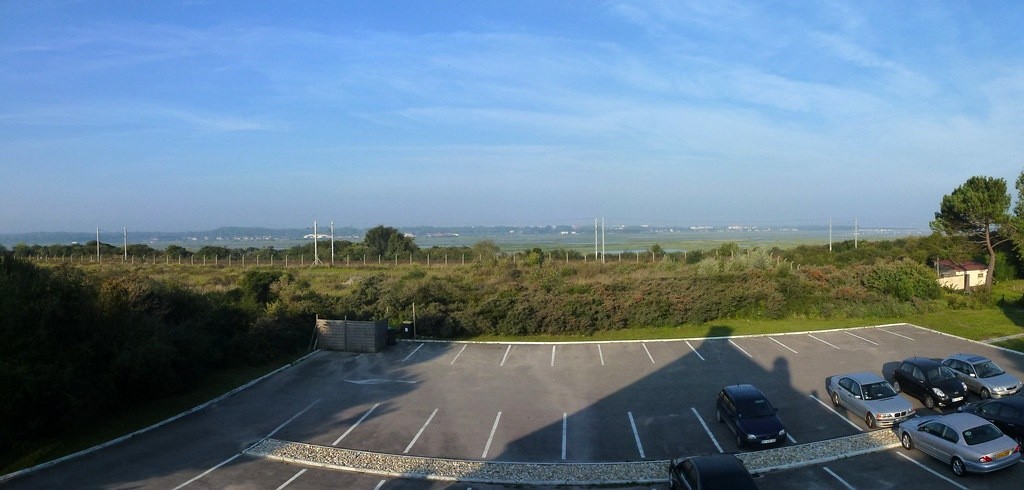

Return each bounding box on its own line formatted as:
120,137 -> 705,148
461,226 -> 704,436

899,412 -> 1022,477
941,352 -> 1023,400
669,453 -> 761,490
960,395 -> 1024,453
893,356 -> 970,409
717,383 -> 787,450
829,371 -> 917,428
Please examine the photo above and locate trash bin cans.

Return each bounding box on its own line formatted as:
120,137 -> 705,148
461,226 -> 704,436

388,327 -> 397,346
402,320 -> 413,339
